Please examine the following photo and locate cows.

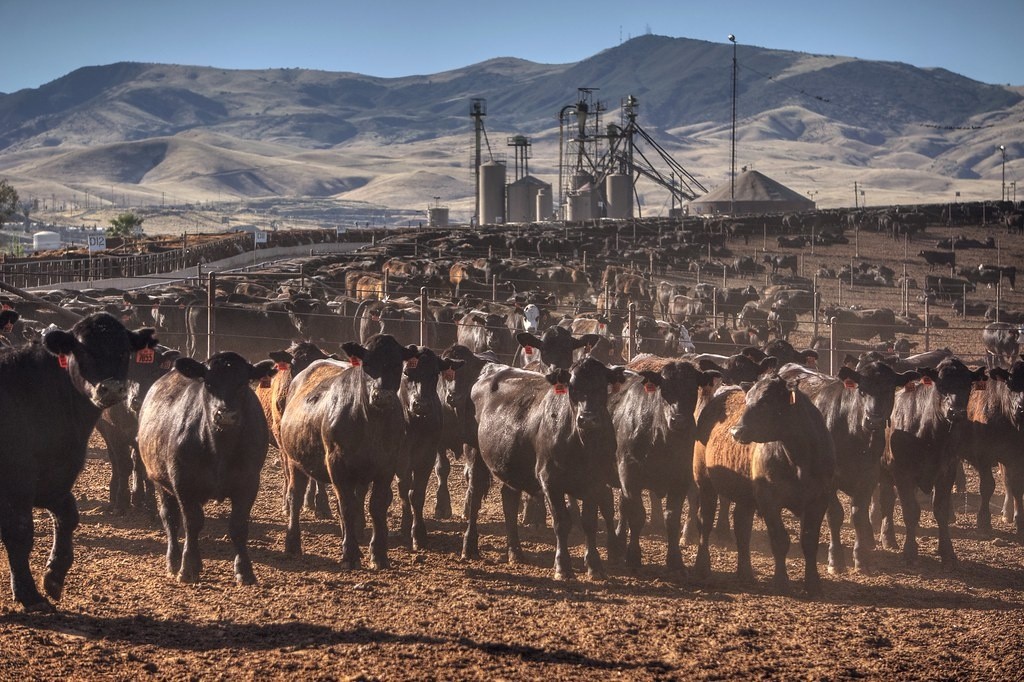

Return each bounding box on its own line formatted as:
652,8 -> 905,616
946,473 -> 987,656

0,200 -> 1022,616
